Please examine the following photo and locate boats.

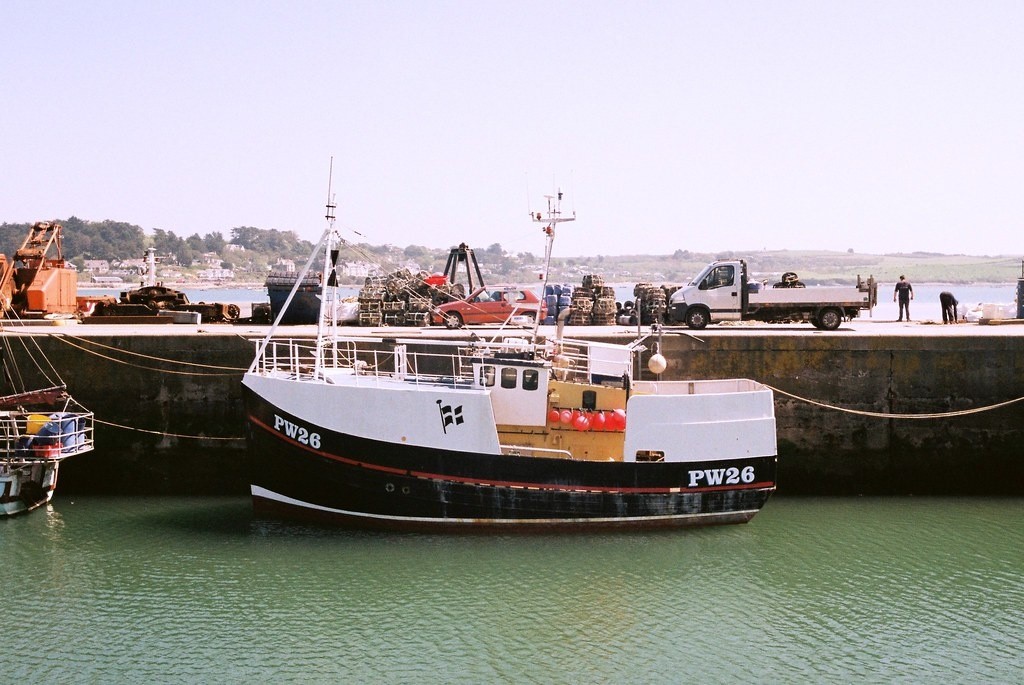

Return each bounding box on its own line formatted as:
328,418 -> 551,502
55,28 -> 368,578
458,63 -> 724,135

0,347 -> 95,520
237,154 -> 780,536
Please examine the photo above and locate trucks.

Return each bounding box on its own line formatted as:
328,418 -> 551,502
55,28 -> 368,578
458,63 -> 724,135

666,258 -> 878,330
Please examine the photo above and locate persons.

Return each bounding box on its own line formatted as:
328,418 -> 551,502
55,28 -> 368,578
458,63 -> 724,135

894,275 -> 913,322
940,291 -> 960,324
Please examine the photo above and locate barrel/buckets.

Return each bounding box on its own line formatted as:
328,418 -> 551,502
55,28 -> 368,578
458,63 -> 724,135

542,284 -> 573,325
15,413 -> 86,456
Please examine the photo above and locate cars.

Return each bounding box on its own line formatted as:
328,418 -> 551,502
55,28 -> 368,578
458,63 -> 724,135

428,284 -> 548,330
